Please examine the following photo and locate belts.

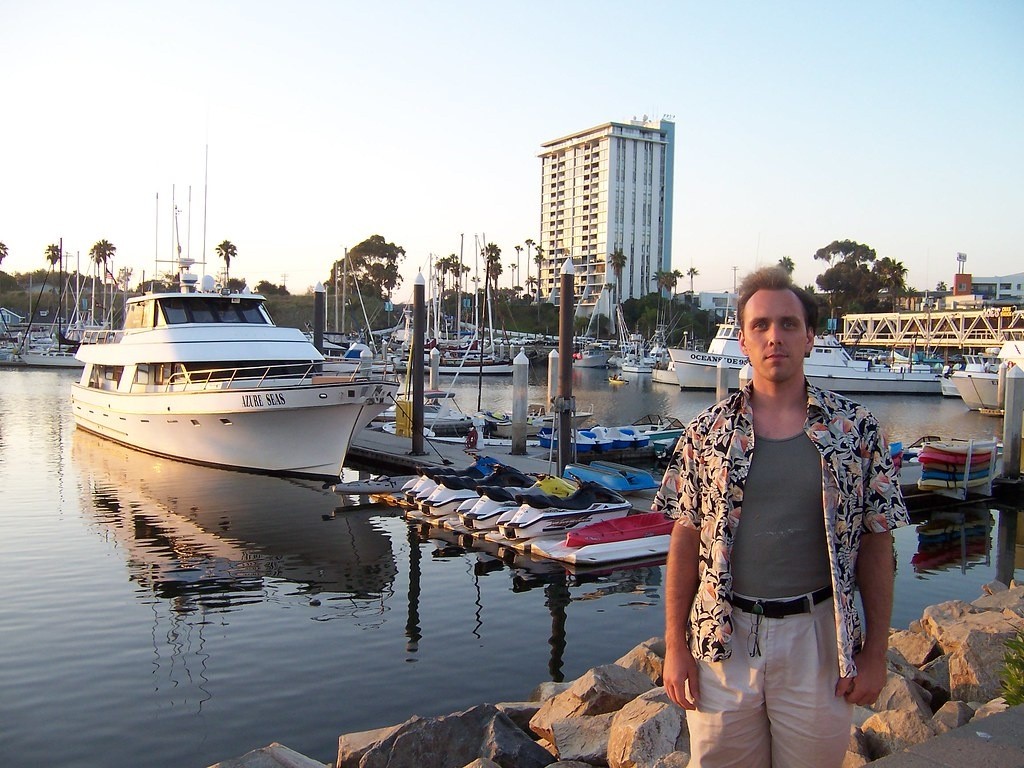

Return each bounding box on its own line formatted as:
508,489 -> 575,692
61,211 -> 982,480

732,586 -> 833,619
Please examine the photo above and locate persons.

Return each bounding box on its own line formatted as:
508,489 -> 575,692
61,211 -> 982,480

878,353 -> 882,363
617,374 -> 623,380
650,268 -> 912,768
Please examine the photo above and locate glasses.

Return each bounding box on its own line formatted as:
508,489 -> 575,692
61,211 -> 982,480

746,599 -> 765,658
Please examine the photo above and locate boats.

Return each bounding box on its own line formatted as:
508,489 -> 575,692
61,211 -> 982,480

574,350 -> 606,369
331,474 -> 420,492
634,414 -> 685,459
608,376 -> 630,386
591,426 -> 650,449
563,508 -> 675,547
477,409 -> 542,437
941,354 -> 1006,416
506,406 -> 594,429
622,321 -> 658,373
669,309 -> 942,396
538,427 -> 613,452
562,460 -> 660,495
71,256 -> 401,484
376,390 -> 474,436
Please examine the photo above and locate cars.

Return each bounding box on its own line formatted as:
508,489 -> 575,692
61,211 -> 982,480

598,342 -> 610,350
573,337 -> 595,345
619,342 -> 631,349
493,337 -> 555,345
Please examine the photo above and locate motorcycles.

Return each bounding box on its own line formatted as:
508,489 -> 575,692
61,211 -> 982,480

456,472 -> 576,530
494,472 -> 633,541
415,460 -> 536,518
401,448 -> 505,505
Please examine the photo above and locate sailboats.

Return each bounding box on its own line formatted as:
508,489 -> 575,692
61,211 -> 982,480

373,232 -> 515,377
646,311 -> 671,369
20,250 -> 87,369
